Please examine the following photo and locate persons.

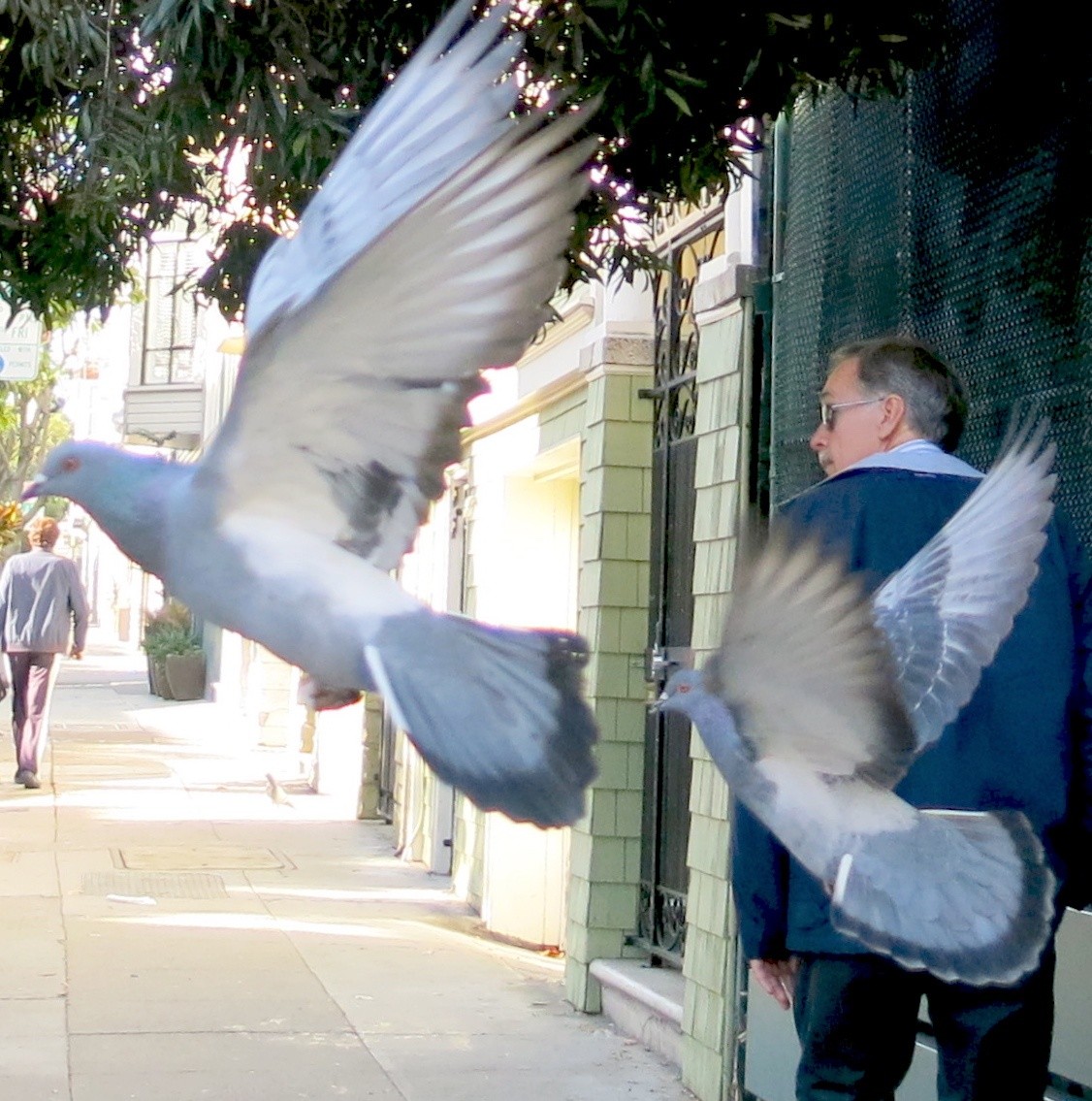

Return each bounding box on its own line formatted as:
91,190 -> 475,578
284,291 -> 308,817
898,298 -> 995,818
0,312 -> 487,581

726,338 -> 1092,1100
0,517 -> 89,788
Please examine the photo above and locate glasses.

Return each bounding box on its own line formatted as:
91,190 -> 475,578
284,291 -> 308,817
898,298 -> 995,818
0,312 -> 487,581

822,398 -> 883,423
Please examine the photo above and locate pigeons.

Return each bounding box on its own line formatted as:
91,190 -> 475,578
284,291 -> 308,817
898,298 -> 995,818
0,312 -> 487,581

650,401 -> 1059,987
15,1 -> 604,830
264,774 -> 294,807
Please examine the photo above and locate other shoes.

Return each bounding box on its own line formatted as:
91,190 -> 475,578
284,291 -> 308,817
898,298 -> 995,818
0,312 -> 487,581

15,770 -> 41,788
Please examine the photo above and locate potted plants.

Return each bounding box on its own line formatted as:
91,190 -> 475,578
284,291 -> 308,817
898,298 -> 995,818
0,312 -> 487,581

140,601 -> 206,700
